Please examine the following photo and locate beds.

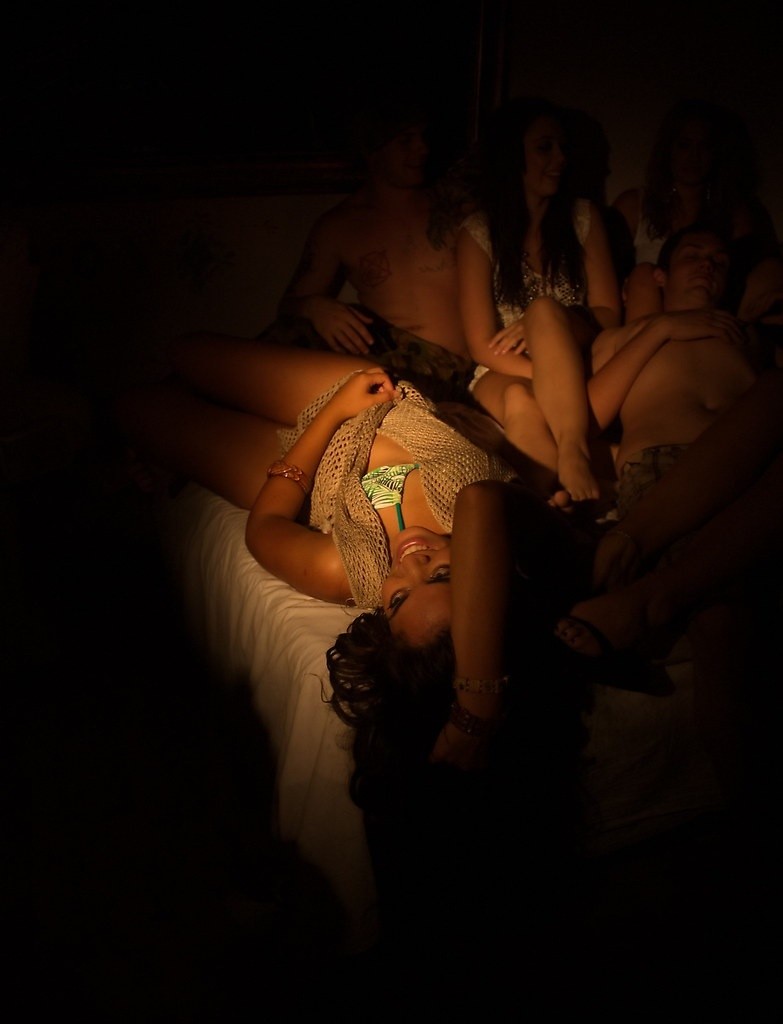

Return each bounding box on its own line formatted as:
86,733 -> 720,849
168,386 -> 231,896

59,193 -> 783,952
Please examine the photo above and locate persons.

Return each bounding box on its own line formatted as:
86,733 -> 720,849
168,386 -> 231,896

108,91 -> 783,775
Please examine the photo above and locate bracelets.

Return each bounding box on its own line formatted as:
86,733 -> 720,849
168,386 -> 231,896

448,703 -> 503,739
608,526 -> 641,553
452,676 -> 509,699
267,459 -> 314,494
